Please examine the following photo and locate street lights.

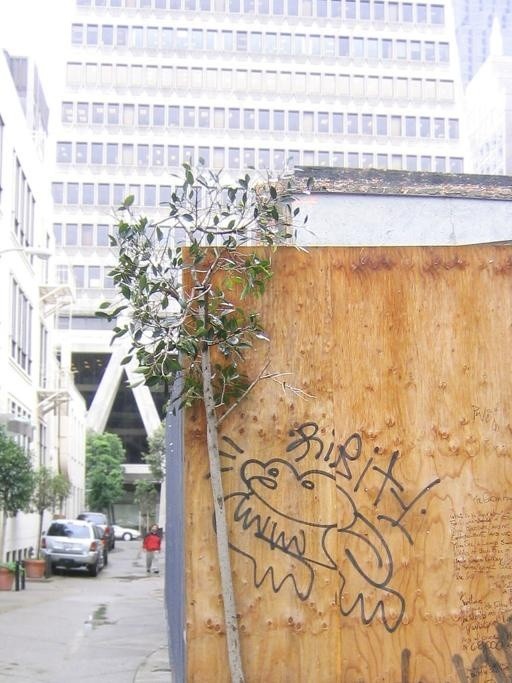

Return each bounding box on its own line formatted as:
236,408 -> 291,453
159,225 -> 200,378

0,247 -> 50,260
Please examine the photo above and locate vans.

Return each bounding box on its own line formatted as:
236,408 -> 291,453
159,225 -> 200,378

40,513 -> 141,576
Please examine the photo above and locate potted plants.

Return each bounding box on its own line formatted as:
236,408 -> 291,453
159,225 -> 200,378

1,424 -> 67,590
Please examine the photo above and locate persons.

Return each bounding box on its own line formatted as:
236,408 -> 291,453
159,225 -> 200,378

142,525 -> 163,573
